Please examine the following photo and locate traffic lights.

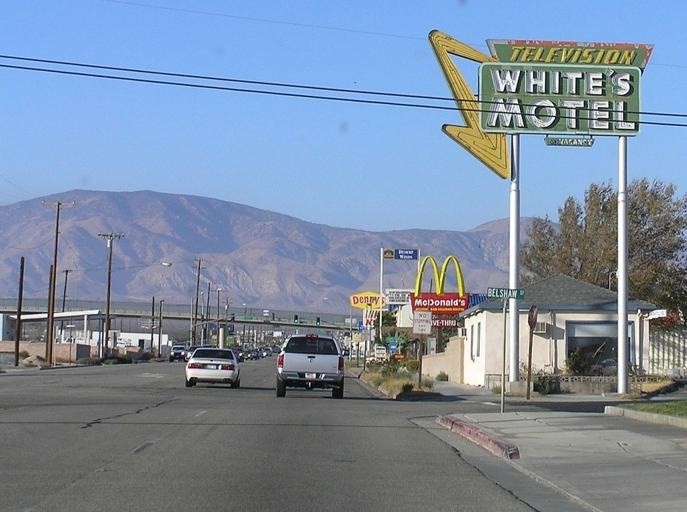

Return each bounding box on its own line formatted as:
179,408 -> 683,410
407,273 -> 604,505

293,314 -> 298,324
316,316 -> 320,326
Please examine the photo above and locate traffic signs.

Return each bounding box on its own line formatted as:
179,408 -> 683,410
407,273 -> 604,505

486,287 -> 524,298
412,310 -> 432,336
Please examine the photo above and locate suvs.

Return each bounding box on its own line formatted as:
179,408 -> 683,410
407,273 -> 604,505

276,335 -> 344,399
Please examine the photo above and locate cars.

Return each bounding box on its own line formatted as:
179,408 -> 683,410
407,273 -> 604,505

172,344 -> 244,386
593,359 -> 618,375
243,345 -> 281,360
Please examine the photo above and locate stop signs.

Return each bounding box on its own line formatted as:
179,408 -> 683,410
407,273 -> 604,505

528,305 -> 538,329
